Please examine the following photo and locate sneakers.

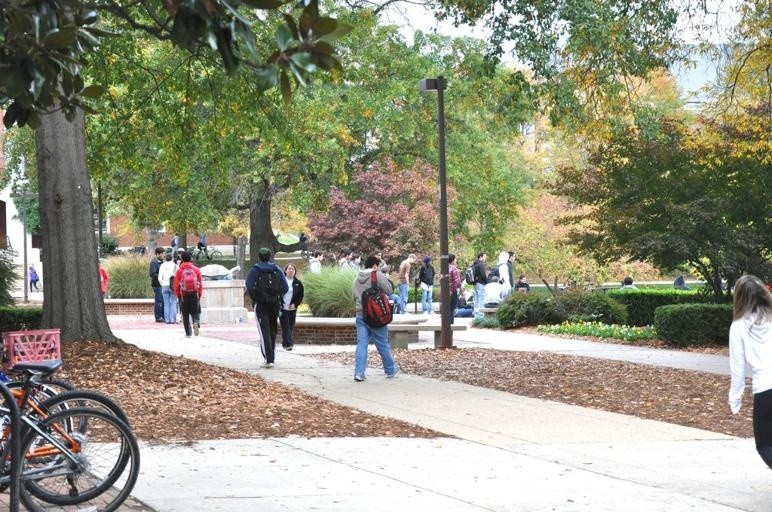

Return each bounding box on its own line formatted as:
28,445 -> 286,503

386,366 -> 400,379
259,358 -> 275,369
184,323 -> 199,338
282,344 -> 294,351
155,314 -> 179,325
354,373 -> 367,382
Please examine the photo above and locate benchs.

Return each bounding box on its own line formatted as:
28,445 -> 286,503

387,323 -> 467,351
473,306 -> 499,317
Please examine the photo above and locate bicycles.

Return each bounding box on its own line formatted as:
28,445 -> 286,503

170,245 -> 223,261
0,357 -> 141,512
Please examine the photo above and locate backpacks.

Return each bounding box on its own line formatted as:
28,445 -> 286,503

361,270 -> 393,328
180,268 -> 199,293
487,262 -> 505,285
465,265 -> 476,285
252,269 -> 281,303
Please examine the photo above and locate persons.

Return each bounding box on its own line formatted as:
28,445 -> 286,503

150,248 -> 202,337
29,267 -> 39,293
279,263 -> 303,351
246,248 -> 288,367
352,257 -> 399,381
620,277 -> 638,290
196,233 -> 213,260
98,261 -> 108,299
309,249 -> 530,314
674,276 -> 690,290
173,233 -> 180,249
728,274 -> 772,469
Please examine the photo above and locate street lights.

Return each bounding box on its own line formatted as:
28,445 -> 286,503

418,73 -> 454,352
9,185 -> 39,301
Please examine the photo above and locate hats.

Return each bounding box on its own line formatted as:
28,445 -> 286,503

423,256 -> 432,264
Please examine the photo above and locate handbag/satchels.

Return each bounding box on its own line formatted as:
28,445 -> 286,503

169,276 -> 175,290
